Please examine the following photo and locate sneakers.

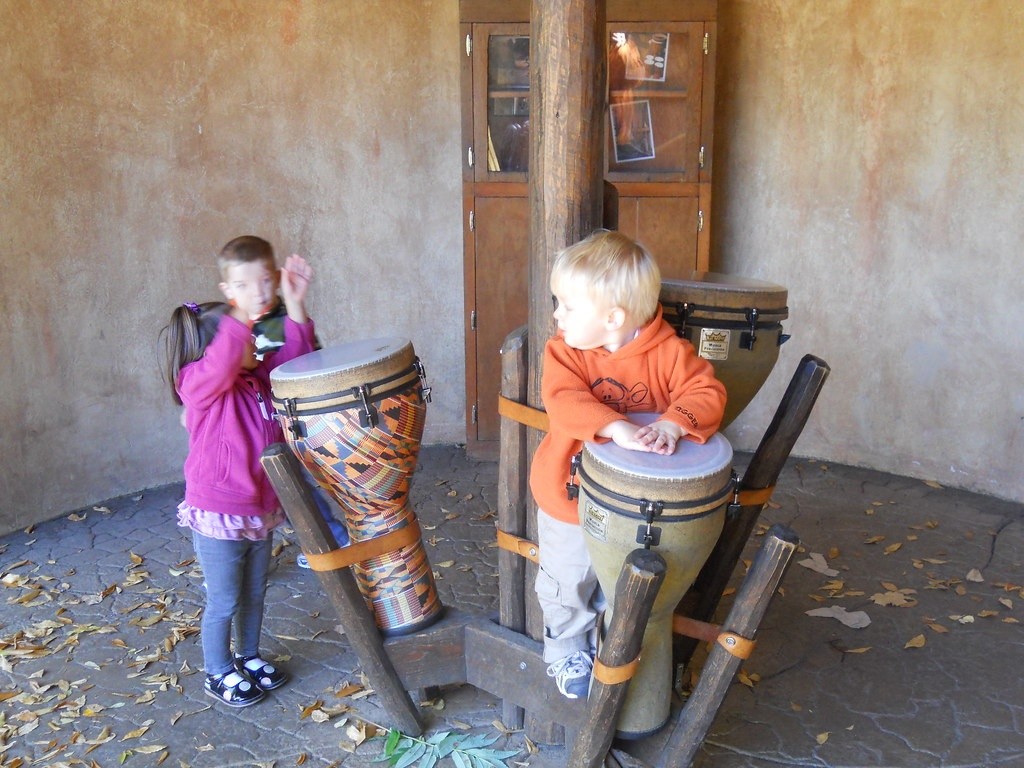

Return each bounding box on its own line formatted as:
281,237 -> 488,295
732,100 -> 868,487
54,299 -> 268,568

297,544 -> 352,569
553,653 -> 592,701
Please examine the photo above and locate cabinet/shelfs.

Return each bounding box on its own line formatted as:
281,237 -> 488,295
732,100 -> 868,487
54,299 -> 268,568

458,0 -> 719,461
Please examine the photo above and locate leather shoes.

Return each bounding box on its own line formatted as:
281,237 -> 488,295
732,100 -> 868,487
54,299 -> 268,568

233,653 -> 288,689
206,669 -> 266,709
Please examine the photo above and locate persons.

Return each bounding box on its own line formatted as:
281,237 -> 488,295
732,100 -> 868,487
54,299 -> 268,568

212,231 -> 354,570
155,253 -> 324,709
529,228 -> 728,698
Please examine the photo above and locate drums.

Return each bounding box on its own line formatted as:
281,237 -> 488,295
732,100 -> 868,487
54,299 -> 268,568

658,270 -> 792,435
578,411 -> 738,739
269,336 -> 446,637
